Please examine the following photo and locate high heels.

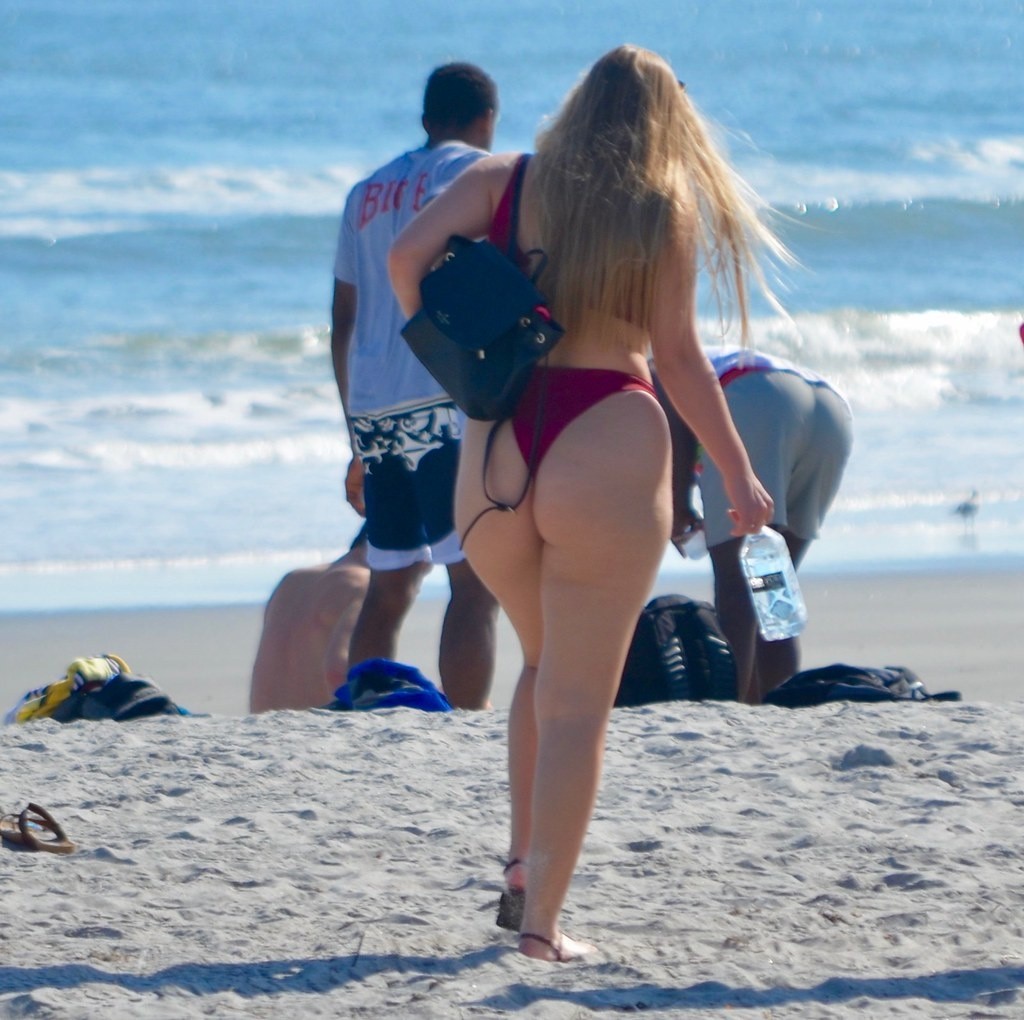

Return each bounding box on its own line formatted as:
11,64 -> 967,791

521,934 -> 599,964
496,859 -> 526,932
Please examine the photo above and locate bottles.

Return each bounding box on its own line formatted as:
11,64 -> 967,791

740,524 -> 807,642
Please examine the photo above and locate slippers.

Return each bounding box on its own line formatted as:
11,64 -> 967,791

0,803 -> 73,853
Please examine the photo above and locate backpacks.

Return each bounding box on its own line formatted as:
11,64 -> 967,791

399,152 -> 566,513
620,595 -> 738,705
763,664 -> 964,708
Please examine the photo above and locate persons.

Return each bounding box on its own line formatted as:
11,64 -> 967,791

250,517 -> 372,715
331,61 -> 500,708
649,348 -> 852,703
387,42 -> 773,962
